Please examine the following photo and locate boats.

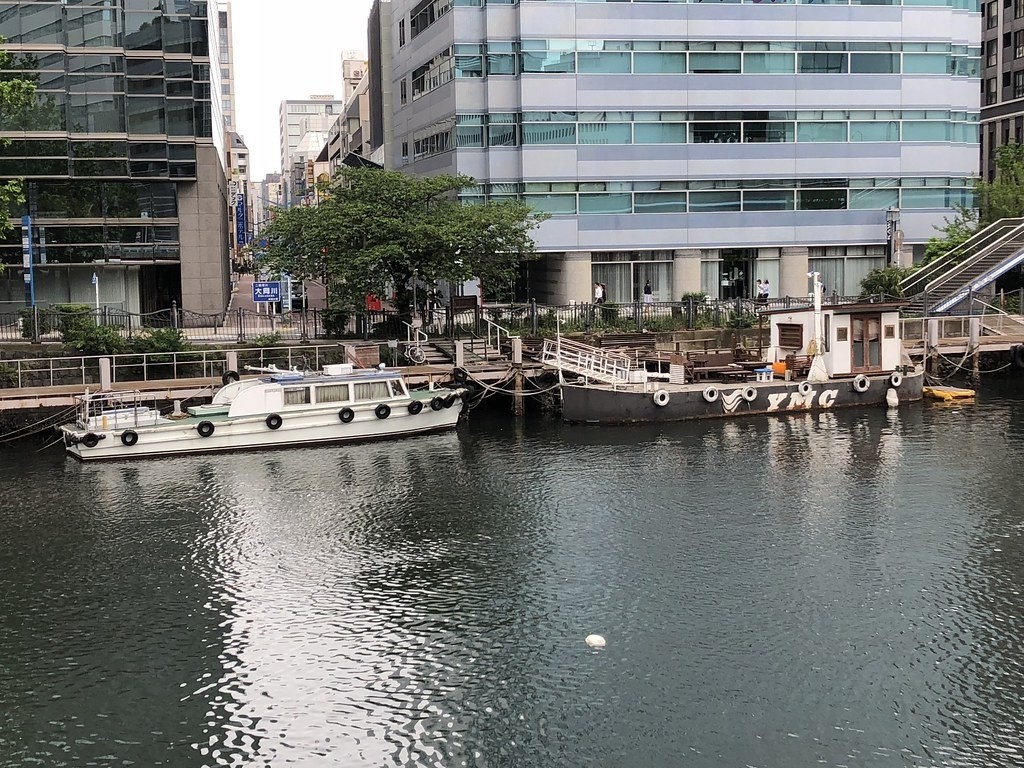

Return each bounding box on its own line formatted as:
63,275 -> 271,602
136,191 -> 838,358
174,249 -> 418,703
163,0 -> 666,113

55,362 -> 471,463
559,298 -> 928,429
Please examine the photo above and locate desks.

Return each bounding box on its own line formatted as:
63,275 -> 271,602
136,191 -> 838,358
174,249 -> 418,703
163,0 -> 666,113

717,371 -> 752,384
754,368 -> 774,382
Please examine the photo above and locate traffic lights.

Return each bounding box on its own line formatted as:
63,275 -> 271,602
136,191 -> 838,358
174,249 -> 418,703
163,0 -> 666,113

321,239 -> 328,254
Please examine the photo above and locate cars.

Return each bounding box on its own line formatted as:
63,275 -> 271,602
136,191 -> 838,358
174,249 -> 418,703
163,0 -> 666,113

269,278 -> 309,314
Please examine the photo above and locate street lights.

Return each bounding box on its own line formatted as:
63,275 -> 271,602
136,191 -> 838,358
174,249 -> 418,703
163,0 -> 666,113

90,272 -> 101,327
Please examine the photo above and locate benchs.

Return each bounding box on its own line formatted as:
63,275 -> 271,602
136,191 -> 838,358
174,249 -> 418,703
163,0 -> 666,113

696,352 -> 744,380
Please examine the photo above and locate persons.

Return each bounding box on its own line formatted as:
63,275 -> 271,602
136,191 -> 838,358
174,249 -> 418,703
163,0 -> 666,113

643,279 -> 655,302
755,278 -> 771,298
592,281 -> 608,304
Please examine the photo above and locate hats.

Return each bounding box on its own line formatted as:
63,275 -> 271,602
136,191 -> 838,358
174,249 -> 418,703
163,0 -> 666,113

764,280 -> 769,284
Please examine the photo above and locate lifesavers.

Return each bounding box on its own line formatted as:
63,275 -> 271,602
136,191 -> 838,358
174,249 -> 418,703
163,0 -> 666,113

443,394 -> 455,408
408,400 -> 423,415
455,368 -> 468,381
222,371 -> 240,385
703,386 -> 719,402
892,372 -> 902,387
83,433 -> 99,447
339,407 -> 354,423
742,386 -> 757,402
431,397 -> 443,411
654,390 -> 670,406
798,381 -> 812,395
121,430 -> 138,446
197,421 -> 215,437
375,405 -> 391,419
853,374 -> 870,392
266,413 -> 283,430
